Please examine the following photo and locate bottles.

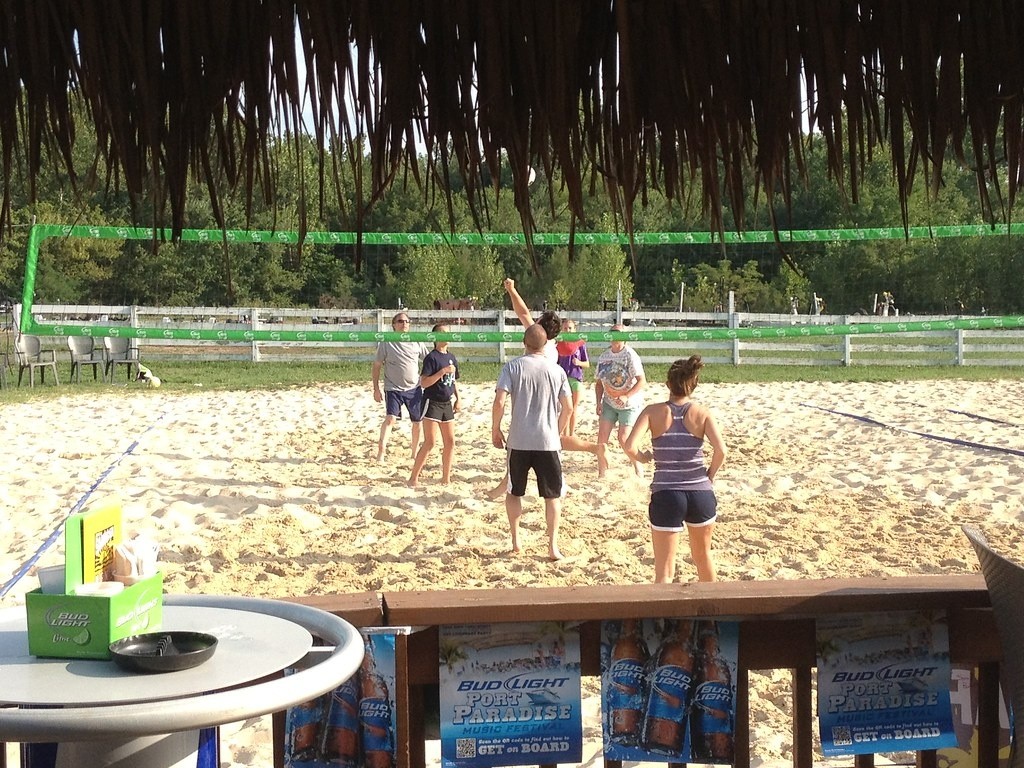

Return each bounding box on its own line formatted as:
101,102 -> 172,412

289,634 -> 395,768
609,619 -> 734,765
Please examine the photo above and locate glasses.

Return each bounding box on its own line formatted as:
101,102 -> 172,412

394,319 -> 410,323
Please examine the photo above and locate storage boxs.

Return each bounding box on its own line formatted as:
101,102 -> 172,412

25,573 -> 165,660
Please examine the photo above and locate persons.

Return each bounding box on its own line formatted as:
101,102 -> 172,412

372,313 -> 430,461
483,277 -> 609,561
407,323 -> 461,487
594,324 -> 646,478
624,355 -> 725,583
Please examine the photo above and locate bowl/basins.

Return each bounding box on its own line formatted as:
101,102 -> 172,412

108,631 -> 218,672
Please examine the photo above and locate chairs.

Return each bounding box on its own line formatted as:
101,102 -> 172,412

14,334 -> 60,389
66,336 -> 107,384
960,524 -> 1024,768
103,336 -> 140,384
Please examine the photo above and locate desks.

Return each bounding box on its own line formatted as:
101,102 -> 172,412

0,592 -> 365,768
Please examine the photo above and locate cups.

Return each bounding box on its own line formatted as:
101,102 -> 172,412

75,582 -> 124,597
37,564 -> 66,595
